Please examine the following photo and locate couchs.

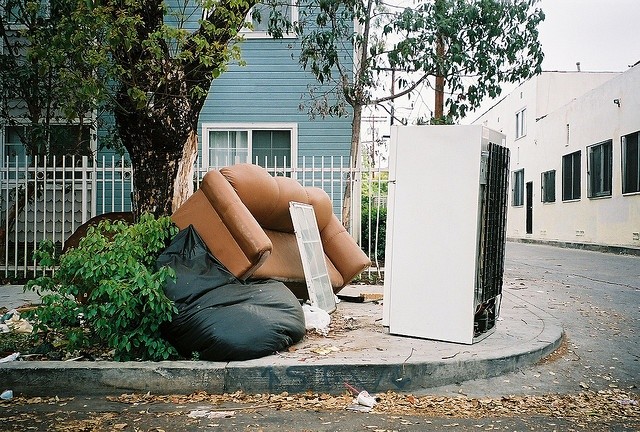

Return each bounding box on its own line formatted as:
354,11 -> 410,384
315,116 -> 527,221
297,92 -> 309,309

168,162 -> 372,295
